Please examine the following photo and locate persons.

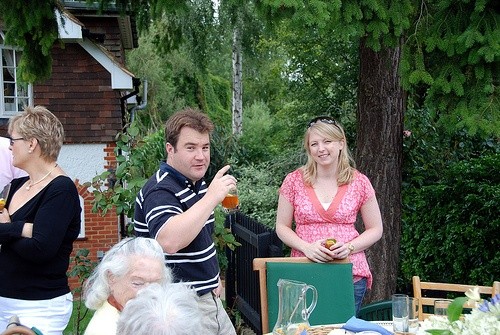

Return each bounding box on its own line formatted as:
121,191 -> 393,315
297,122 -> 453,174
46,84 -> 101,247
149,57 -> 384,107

116,279 -> 202,335
275,117 -> 384,318
0,106 -> 83,335
133,106 -> 238,335
81,236 -> 174,335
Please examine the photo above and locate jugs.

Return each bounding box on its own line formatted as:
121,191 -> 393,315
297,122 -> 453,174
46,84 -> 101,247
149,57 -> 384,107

273,279 -> 318,335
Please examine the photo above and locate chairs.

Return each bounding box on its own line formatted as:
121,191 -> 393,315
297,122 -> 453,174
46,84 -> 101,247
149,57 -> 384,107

413,276 -> 500,321
253,256 -> 356,334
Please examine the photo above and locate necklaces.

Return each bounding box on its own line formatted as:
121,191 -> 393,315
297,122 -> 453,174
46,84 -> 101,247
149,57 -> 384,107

25,164 -> 58,191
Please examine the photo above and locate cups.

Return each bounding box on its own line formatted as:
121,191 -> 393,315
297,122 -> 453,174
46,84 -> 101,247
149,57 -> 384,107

410,297 -> 419,333
391,293 -> 410,334
434,300 -> 451,317
324,239 -> 336,248
0,199 -> 7,213
222,186 -> 237,209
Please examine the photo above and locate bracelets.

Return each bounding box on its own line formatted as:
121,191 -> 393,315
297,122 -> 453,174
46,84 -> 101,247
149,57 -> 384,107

347,242 -> 354,256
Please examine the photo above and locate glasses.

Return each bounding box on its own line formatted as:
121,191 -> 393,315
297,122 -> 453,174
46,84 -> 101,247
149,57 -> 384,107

307,116 -> 342,134
9,136 -> 24,146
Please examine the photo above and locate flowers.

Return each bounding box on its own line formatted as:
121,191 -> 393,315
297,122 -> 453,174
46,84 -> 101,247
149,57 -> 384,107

424,285 -> 500,335
